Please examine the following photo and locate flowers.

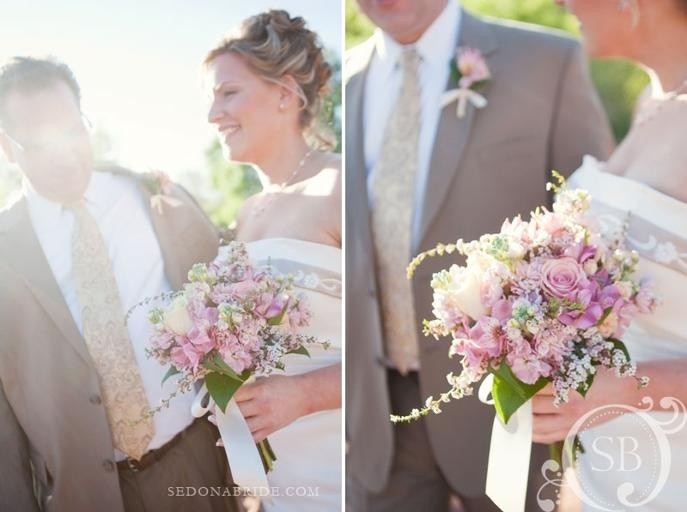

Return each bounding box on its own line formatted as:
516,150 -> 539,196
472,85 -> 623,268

112,239 -> 334,477
437,47 -> 495,116
142,170 -> 181,212
390,170 -> 663,473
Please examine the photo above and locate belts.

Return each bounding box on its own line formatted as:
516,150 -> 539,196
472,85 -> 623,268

114,411 -> 210,475
388,368 -> 418,381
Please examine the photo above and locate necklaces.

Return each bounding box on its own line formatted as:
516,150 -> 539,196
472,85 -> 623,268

632,80 -> 685,124
244,143 -> 313,223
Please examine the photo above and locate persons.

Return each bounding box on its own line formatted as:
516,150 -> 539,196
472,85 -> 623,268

527,1 -> 685,510
0,52 -> 239,510
203,8 -> 341,512
344,0 -> 621,511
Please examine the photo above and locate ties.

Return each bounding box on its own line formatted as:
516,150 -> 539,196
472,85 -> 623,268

60,190 -> 156,462
366,44 -> 424,378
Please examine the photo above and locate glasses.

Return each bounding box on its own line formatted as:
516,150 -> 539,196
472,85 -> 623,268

5,112 -> 95,160
